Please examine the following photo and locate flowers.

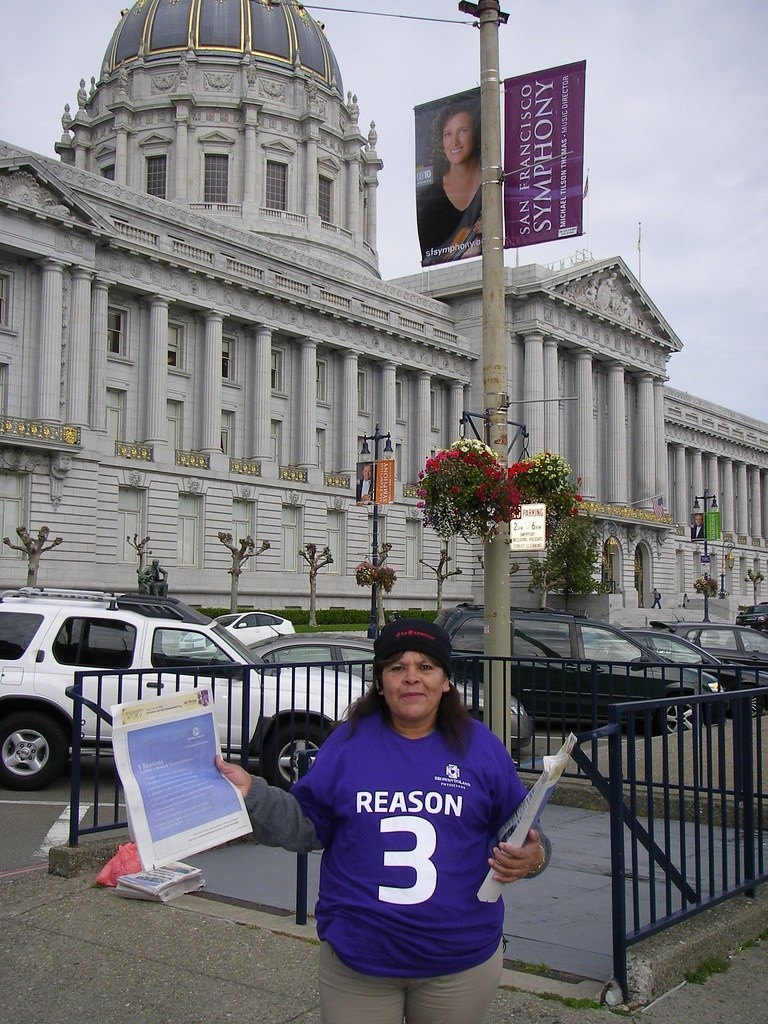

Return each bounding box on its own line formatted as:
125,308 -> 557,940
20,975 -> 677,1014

508,452 -> 583,538
414,440 -> 519,537
693,579 -> 719,598
356,564 -> 397,594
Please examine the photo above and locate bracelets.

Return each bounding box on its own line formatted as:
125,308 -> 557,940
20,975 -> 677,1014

530,846 -> 545,873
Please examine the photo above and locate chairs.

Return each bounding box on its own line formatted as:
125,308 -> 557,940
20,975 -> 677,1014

137,578 -> 168,597
704,633 -> 721,647
724,637 -> 737,649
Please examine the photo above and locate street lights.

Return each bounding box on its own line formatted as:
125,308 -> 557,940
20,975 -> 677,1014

357,422 -> 393,638
692,487 -> 718,623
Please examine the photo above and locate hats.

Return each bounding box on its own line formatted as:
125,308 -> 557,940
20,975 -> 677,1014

373,618 -> 455,678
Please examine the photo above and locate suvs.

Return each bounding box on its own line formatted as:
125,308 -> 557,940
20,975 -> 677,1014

432,602 -> 729,735
0,591 -> 369,794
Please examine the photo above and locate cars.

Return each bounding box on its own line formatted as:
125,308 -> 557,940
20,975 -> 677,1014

211,611 -> 296,647
649,620 -> 768,666
735,601 -> 768,633
251,631 -> 534,751
619,630 -> 768,717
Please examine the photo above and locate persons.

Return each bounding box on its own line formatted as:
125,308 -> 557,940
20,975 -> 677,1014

215,618 -> 551,1022
683,593 -> 688,609
356,465 -> 373,505
418,104 -> 485,264
650,588 -> 662,610
